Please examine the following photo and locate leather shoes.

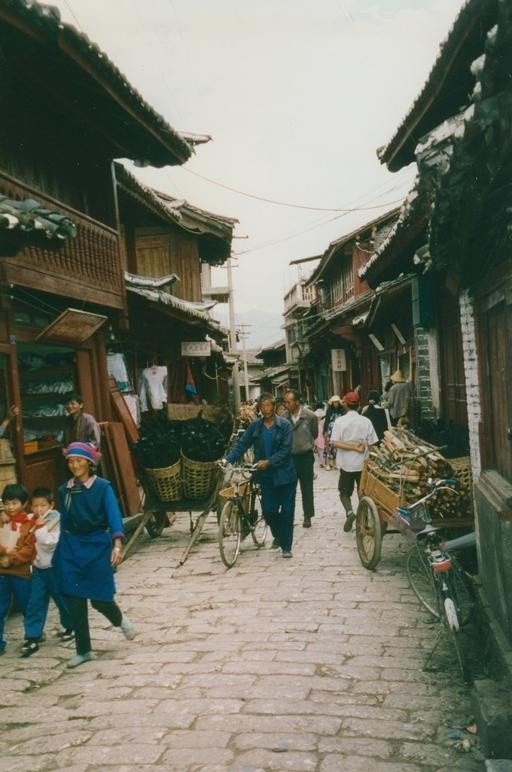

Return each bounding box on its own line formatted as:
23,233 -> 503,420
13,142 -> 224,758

303,518 -> 310,527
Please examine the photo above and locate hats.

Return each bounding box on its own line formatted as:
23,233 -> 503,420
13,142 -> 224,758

344,393 -> 360,404
328,395 -> 341,404
390,370 -> 405,382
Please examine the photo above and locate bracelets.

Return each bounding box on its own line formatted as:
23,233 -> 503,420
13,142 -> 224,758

112,546 -> 119,552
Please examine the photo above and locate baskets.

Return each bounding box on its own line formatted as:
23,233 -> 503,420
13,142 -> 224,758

180,448 -> 221,500
137,453 -> 181,502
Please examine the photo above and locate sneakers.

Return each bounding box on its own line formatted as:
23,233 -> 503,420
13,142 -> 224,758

121,614 -> 134,640
272,537 -> 281,549
67,652 -> 90,668
283,550 -> 292,558
343,514 -> 356,532
61,628 -> 75,640
0,641 -> 8,656
19,643 -> 38,654
38,631 -> 47,642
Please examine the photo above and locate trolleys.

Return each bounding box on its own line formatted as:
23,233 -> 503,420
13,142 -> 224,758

117,487 -> 217,565
356,461 -> 474,569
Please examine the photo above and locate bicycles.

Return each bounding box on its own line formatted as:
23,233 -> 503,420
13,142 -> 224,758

397,480 -> 474,680
215,461 -> 268,567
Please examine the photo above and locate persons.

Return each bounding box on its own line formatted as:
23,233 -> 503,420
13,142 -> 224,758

213,392 -> 298,558
62,393 -> 104,479
383,369 -> 413,426
0,484 -> 46,656
18,486 -> 76,655
241,399 -> 260,416
312,403 -> 327,468
280,389 -> 318,528
330,392 -> 379,531
361,390 -> 391,444
0,405 -> 20,439
48,442 -> 137,669
276,401 -> 286,417
321,394 -> 346,471
